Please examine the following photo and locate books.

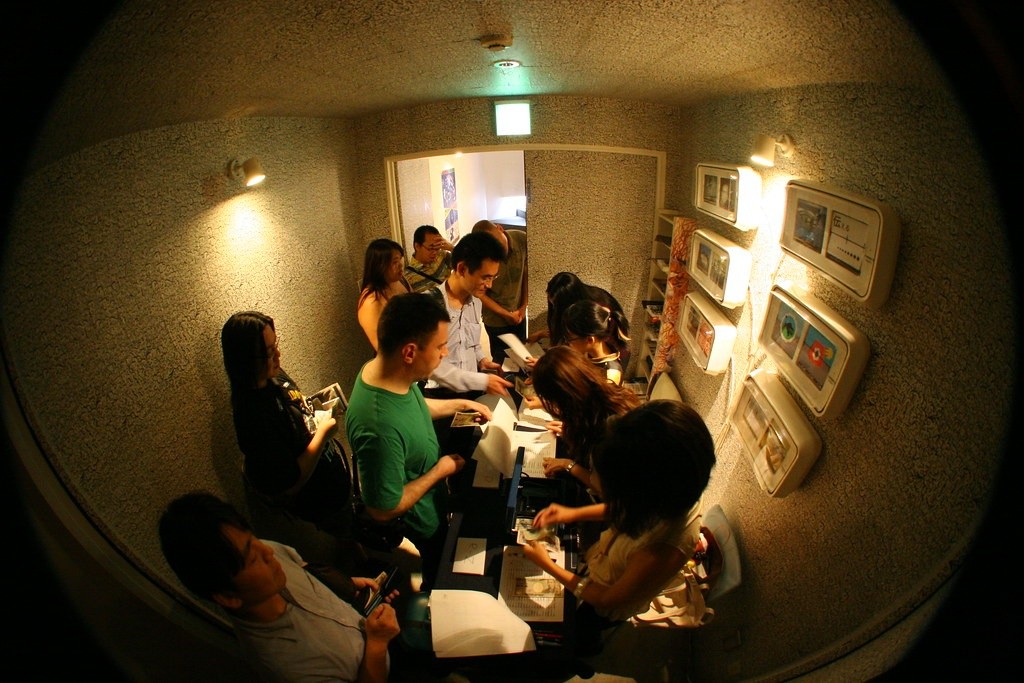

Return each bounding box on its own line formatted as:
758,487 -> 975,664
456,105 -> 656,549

471,398 -> 557,479
497,333 -> 546,374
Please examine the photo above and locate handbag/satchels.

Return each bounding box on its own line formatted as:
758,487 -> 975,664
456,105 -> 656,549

352,494 -> 403,550
624,539 -> 714,629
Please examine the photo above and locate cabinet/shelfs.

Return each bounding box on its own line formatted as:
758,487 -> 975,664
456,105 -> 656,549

636,210 -> 694,391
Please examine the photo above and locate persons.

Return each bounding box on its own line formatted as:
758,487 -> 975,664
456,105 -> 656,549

524,398 -> 716,656
563,299 -> 632,386
221,310 -> 364,553
158,492 -> 401,683
472,219 -> 528,364
357,238 -> 414,352
532,345 -> 643,494
403,225 -> 455,293
546,272 -> 631,385
418,231 -> 514,498
345,293 -> 492,591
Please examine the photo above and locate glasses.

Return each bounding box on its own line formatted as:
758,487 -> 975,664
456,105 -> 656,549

467,266 -> 500,284
563,333 -> 586,344
267,336 -> 280,358
420,244 -> 442,253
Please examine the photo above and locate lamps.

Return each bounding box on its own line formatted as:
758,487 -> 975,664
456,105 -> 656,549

232,160 -> 265,186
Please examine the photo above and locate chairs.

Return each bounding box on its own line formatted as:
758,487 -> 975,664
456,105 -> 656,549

604,502 -> 746,641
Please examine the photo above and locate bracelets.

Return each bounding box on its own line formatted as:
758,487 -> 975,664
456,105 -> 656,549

566,461 -> 576,472
574,576 -> 592,597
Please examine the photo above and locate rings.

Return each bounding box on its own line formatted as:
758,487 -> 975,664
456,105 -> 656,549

442,243 -> 444,246
